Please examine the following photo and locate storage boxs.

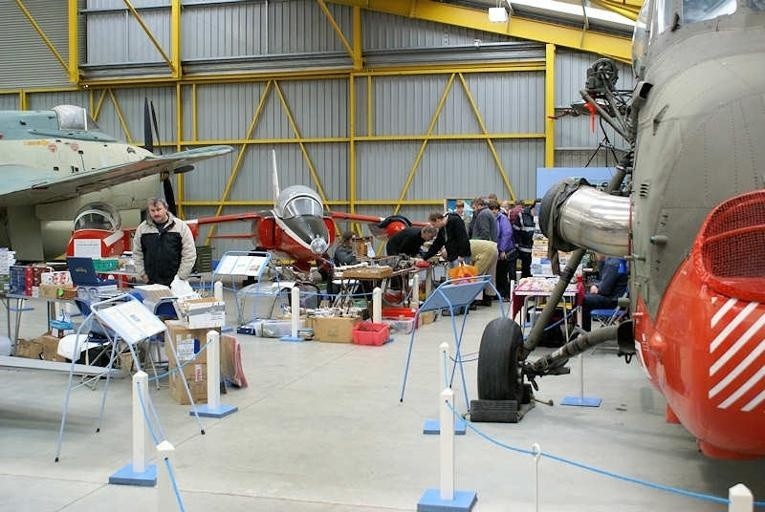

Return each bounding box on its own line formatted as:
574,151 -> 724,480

14,318 -> 74,362
38,282 -> 76,301
246,306 -> 435,347
163,319 -> 222,407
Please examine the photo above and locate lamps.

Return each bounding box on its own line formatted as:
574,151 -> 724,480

488,0 -> 511,23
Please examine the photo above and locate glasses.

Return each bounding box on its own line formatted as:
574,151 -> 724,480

352,238 -> 356,242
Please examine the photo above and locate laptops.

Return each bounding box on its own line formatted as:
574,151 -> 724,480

66,256 -> 118,285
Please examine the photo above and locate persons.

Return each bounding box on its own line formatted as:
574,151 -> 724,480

415,212 -> 472,313
453,192 -> 543,302
334,232 -> 382,301
132,195 -> 197,290
582,253 -> 628,331
386,222 -> 437,290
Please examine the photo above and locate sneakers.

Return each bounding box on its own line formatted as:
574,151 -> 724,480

469,296 -> 491,311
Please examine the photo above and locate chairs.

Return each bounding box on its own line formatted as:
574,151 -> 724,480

136,297 -> 182,389
589,287 -> 630,334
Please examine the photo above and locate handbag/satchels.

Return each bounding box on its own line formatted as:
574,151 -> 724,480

448,265 -> 479,285
504,247 -> 519,261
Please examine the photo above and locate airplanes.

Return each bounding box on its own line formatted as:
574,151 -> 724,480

181,149 -> 433,275
477,0 -> 765,463
1,104 -> 236,269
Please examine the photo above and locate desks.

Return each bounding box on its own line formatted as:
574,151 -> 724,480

337,252 -> 446,325
3,284 -> 169,359
508,269 -> 583,344
94,269 -> 142,290
